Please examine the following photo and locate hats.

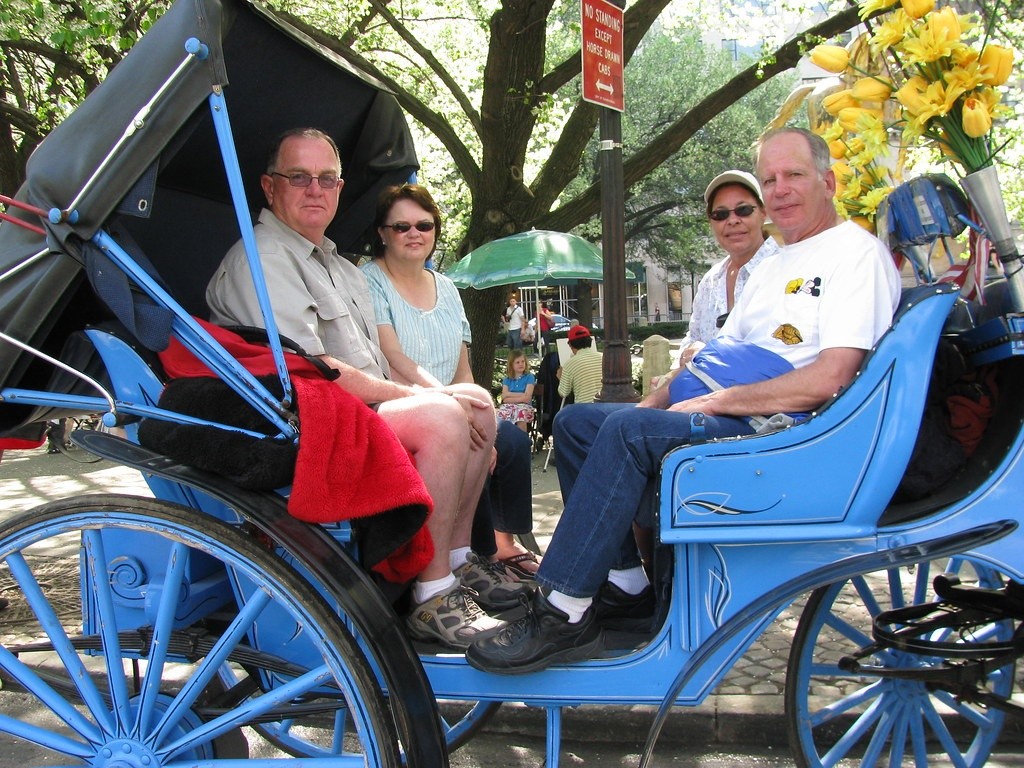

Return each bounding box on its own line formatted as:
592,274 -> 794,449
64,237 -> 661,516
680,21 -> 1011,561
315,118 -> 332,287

704,170 -> 764,205
567,324 -> 591,342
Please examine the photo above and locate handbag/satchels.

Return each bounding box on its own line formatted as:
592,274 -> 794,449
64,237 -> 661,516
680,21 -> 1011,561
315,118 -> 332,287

543,309 -> 555,328
506,315 -> 511,322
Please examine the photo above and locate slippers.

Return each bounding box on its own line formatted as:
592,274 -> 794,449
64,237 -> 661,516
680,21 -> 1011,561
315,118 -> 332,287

502,549 -> 540,579
485,558 -> 529,586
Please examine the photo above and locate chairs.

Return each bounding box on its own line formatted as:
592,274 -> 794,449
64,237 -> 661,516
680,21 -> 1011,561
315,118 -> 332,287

491,384 -> 548,458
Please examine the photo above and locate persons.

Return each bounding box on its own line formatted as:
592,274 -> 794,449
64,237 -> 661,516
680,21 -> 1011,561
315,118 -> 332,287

204,128 -> 540,650
653,303 -> 661,324
533,300 -> 553,355
495,351 -> 535,432
554,325 -> 604,404
461,129 -> 902,676
505,297 -> 525,350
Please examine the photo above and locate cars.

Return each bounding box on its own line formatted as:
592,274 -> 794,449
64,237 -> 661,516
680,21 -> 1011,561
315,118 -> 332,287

527,315 -> 570,333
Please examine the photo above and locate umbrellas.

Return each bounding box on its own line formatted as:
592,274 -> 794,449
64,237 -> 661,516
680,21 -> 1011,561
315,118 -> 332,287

441,225 -> 636,292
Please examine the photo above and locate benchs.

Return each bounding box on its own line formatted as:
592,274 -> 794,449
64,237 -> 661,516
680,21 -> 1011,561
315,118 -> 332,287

651,281 -> 961,544
86,324 -> 365,541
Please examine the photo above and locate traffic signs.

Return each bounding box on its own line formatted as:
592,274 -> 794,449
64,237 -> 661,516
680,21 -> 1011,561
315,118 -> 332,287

581,0 -> 626,113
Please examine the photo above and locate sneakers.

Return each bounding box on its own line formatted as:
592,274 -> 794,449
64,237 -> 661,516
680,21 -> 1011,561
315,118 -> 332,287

451,550 -> 534,611
465,585 -> 608,675
404,575 -> 510,650
598,579 -> 660,651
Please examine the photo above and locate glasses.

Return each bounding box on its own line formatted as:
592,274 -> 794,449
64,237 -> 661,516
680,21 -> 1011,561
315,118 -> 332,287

708,204 -> 763,221
378,221 -> 434,232
270,172 -> 342,188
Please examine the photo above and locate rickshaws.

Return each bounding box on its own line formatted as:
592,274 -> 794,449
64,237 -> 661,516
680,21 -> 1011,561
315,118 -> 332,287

0,0 -> 1023,767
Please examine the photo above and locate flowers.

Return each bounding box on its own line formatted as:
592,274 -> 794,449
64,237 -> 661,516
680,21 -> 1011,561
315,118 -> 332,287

755,0 -> 1024,178
797,98 -> 906,238
